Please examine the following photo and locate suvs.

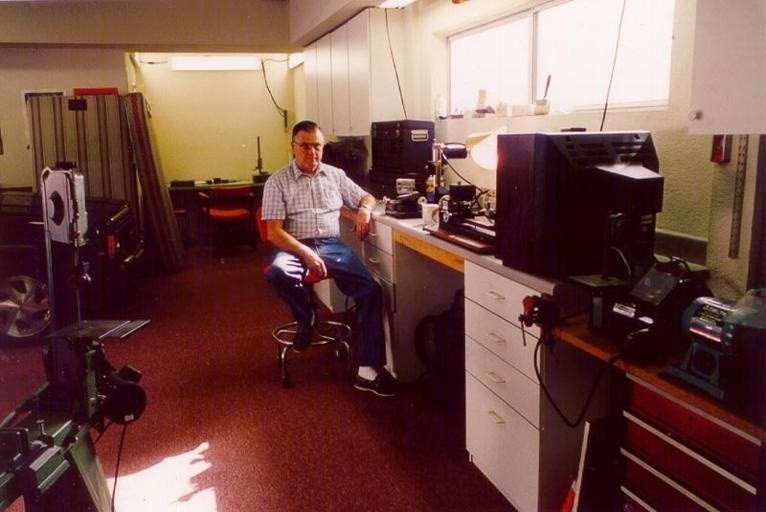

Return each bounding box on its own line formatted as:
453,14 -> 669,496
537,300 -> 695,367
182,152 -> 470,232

1,185 -> 145,351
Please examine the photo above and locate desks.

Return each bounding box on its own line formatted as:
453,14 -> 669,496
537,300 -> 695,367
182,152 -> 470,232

167,180 -> 261,209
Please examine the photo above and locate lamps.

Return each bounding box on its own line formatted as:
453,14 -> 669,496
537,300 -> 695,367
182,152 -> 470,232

442,124 -> 510,171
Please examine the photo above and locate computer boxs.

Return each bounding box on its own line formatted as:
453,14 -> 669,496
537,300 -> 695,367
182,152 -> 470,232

371,120 -> 434,199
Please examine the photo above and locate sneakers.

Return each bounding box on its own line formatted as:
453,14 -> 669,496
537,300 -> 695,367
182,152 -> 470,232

293,310 -> 317,353
353,375 -> 396,396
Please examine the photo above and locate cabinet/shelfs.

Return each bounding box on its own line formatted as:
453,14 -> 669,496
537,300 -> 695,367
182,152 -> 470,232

336,204 -> 766,512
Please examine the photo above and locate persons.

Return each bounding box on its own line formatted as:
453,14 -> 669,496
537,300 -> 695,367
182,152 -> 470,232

260,119 -> 402,402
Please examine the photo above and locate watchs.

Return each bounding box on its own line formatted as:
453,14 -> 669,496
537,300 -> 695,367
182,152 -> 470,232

360,204 -> 372,213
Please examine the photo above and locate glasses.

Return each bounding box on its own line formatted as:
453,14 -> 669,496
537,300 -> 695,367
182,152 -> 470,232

293,141 -> 323,151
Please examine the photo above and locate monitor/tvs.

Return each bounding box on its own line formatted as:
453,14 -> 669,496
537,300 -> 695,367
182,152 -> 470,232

495,130 -> 665,280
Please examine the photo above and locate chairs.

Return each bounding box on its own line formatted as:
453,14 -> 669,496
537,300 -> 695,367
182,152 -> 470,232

197,179 -> 354,388
